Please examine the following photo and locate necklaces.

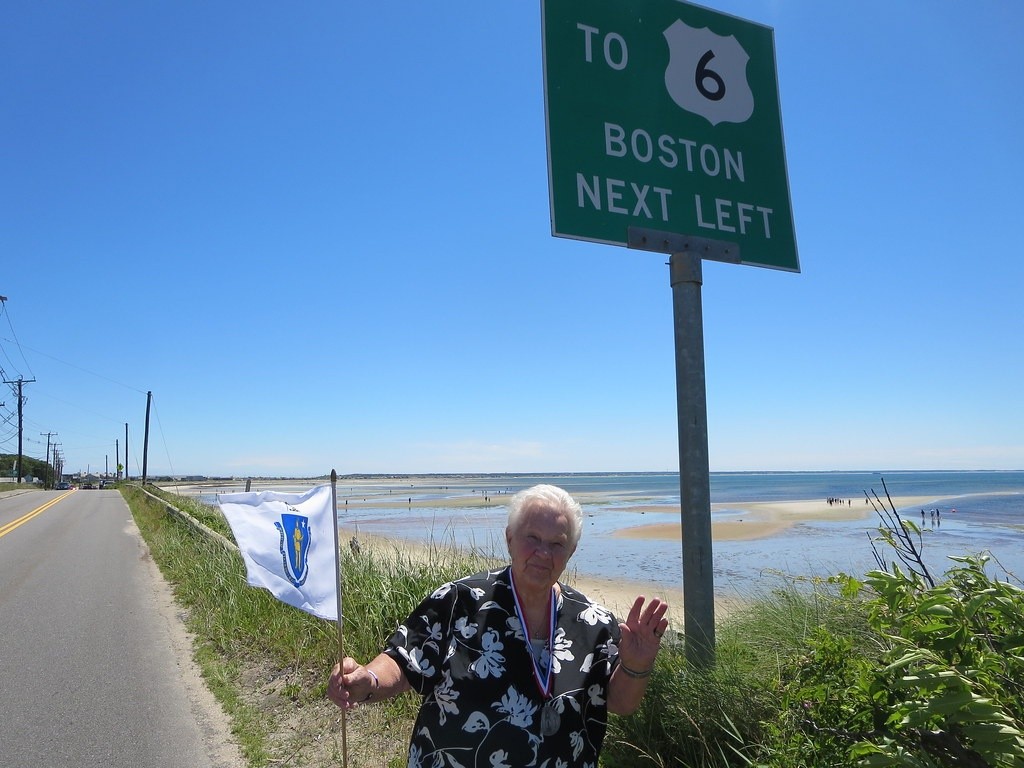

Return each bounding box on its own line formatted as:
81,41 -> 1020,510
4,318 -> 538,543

524,606 -> 548,640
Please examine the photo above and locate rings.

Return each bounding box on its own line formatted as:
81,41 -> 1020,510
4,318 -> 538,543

655,631 -> 663,637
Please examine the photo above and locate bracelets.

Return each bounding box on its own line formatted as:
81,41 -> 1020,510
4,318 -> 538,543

355,670 -> 379,703
619,659 -> 653,679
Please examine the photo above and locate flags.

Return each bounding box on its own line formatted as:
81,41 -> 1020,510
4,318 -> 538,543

215,485 -> 339,621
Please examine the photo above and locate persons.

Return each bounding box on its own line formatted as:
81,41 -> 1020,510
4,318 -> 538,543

326,482 -> 672,768
865,498 -> 869,506
920,508 -> 942,520
826,497 -> 852,508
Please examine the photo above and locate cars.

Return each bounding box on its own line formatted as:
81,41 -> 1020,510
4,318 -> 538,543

69,485 -> 76,490
104,481 -> 116,489
56,482 -> 70,490
83,483 -> 92,489
